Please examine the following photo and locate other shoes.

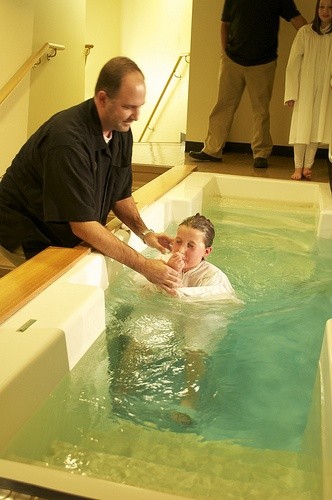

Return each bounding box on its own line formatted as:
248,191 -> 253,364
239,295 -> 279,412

190,150 -> 223,162
253,157 -> 268,168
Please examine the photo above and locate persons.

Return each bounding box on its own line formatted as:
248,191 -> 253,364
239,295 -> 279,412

0,55 -> 183,297
115,213 -> 236,300
283,0 -> 332,180
189,0 -> 307,168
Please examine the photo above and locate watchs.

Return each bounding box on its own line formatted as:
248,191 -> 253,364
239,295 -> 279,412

141,229 -> 155,244
